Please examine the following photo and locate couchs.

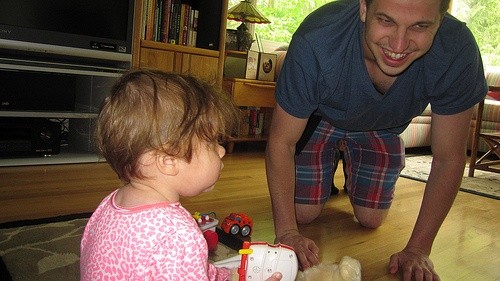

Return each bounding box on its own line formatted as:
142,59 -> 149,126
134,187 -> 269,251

261,43 -> 500,154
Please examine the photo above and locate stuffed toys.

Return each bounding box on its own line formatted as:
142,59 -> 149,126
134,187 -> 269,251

231,255 -> 363,280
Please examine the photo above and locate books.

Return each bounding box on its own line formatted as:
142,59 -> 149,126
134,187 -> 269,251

142,0 -> 197,47
231,106 -> 274,136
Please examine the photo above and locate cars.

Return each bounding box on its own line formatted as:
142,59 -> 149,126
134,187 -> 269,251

220,212 -> 254,237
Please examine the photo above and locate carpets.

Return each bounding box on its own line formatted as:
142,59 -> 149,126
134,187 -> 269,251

0,217 -> 239,281
399,154 -> 500,200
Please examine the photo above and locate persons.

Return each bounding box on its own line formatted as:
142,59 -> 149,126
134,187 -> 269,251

265,0 -> 489,281
79,70 -> 281,281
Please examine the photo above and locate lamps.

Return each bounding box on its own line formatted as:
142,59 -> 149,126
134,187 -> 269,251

226,0 -> 271,52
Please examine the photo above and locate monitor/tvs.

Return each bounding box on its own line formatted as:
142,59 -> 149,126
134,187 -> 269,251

0,0 -> 136,73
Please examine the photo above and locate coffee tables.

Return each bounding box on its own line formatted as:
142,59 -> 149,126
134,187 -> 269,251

469,86 -> 500,177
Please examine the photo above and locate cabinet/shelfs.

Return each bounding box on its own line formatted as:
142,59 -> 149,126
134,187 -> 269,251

132,0 -> 229,89
222,50 -> 277,154
0,63 -> 123,225
